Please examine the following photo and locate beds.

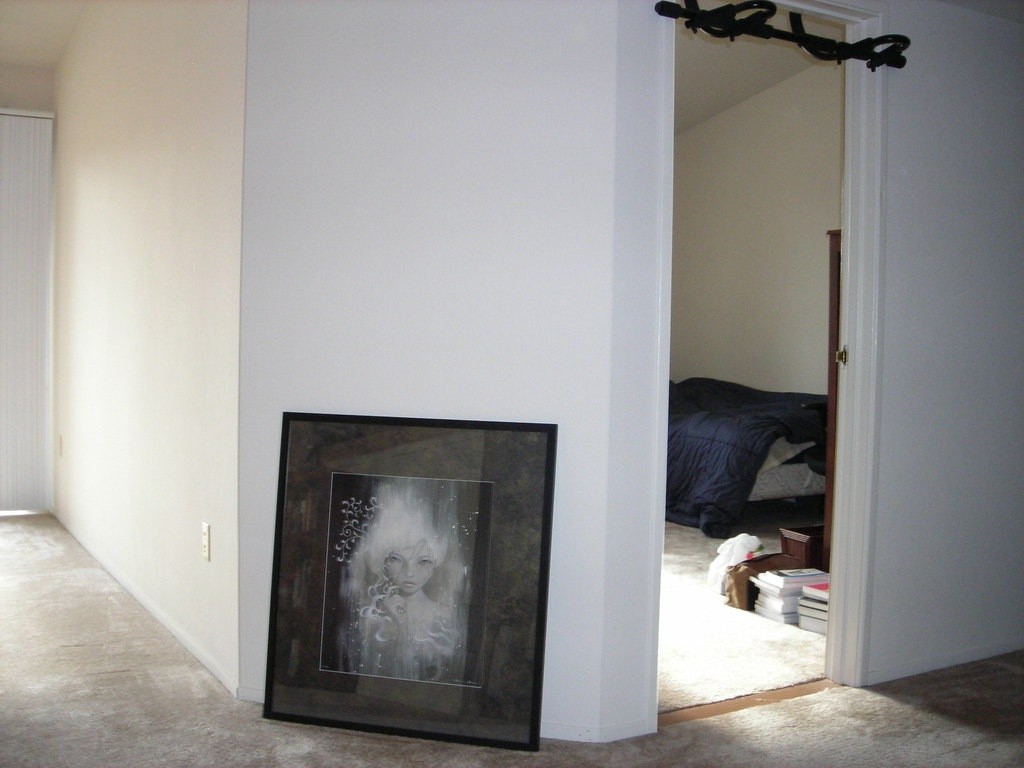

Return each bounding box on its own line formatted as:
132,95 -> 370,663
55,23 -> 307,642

665,377 -> 829,539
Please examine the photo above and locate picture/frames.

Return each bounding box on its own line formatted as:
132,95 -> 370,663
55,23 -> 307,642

263,412 -> 558,752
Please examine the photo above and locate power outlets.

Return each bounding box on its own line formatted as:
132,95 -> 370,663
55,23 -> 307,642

202,522 -> 210,561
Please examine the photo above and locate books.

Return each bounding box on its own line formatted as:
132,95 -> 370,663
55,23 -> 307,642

779,526 -> 825,542
748,568 -> 829,635
